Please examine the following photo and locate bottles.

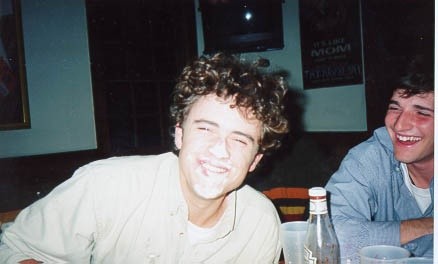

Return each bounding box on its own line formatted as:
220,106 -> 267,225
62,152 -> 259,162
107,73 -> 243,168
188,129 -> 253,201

303,187 -> 341,264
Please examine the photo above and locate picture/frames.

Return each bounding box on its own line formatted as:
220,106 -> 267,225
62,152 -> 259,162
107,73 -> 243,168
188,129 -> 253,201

0,0 -> 31,131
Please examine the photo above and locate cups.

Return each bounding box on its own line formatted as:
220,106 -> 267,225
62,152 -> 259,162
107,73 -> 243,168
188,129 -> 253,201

396,257 -> 433,264
360,245 -> 410,264
279,221 -> 307,264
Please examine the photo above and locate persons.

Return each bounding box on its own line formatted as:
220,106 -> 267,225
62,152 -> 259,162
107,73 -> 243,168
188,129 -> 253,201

0,51 -> 302,264
306,65 -> 434,263
